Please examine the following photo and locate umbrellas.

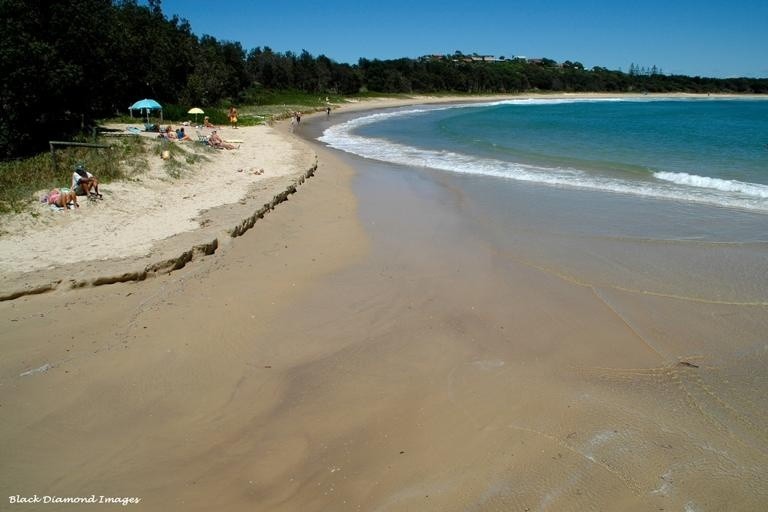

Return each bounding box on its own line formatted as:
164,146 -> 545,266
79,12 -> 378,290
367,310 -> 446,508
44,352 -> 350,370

187,108 -> 205,123
132,98 -> 163,124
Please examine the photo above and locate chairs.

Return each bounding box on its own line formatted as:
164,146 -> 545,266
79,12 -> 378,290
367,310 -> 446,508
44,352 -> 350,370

144,123 -> 164,131
195,130 -> 209,145
126,127 -> 162,137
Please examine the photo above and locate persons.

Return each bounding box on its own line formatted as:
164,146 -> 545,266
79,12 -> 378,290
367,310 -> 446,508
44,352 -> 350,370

327,106 -> 331,115
142,116 -> 160,132
49,188 -> 79,211
229,105 -> 238,129
291,110 -> 301,125
204,116 -> 214,127
71,165 -> 102,200
166,126 -> 240,149
